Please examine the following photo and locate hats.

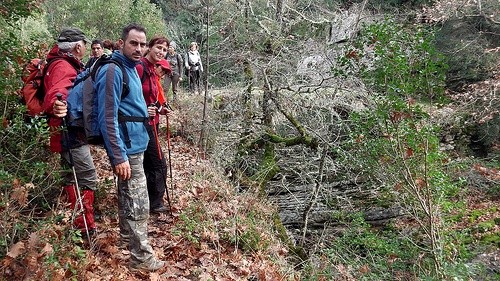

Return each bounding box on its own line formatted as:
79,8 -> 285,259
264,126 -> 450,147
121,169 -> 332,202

155,59 -> 172,73
58,27 -> 92,44
169,41 -> 176,49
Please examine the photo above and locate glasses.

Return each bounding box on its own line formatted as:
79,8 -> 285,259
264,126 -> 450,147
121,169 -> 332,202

162,68 -> 167,74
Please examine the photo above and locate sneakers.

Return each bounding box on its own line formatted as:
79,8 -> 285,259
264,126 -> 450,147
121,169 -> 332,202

150,206 -> 170,214
120,241 -> 130,255
128,257 -> 164,272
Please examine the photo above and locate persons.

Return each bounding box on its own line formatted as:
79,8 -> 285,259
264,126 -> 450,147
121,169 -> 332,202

42,27 -> 102,238
95,23 -> 172,272
134,36 -> 173,214
85,39 -> 204,97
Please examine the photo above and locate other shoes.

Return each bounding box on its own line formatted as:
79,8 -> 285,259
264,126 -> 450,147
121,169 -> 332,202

81,231 -> 112,252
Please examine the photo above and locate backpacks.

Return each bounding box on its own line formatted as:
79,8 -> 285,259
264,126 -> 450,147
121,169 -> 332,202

64,54 -> 150,143
20,59 -> 80,116
